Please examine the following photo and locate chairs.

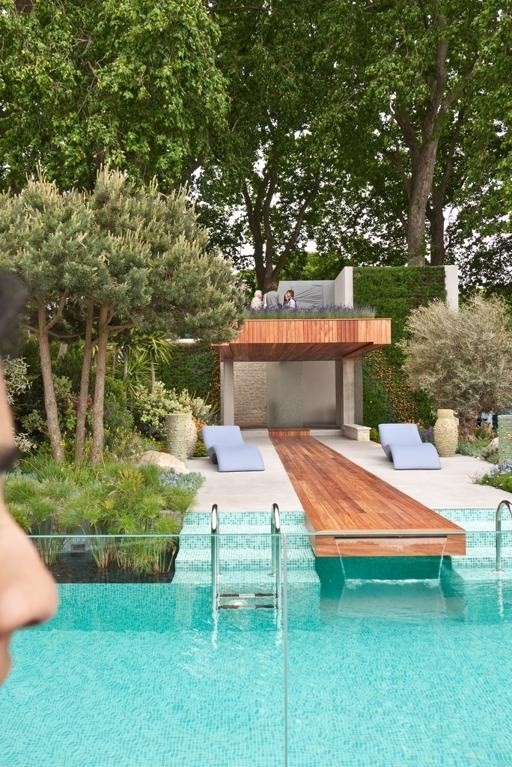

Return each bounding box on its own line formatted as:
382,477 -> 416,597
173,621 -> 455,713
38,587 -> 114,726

379,423 -> 441,470
201,425 -> 264,471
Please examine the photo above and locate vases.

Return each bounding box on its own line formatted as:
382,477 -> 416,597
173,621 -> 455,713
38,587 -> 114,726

433,409 -> 460,457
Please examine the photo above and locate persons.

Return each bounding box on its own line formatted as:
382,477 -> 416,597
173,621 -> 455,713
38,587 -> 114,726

283,290 -> 297,309
251,289 -> 264,309
0,267 -> 59,696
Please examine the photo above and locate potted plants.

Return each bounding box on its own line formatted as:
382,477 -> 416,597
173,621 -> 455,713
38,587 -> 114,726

137,383 -> 211,460
